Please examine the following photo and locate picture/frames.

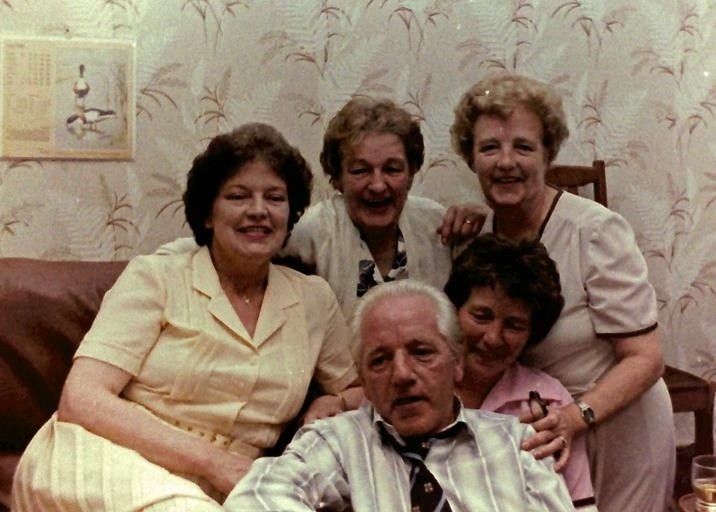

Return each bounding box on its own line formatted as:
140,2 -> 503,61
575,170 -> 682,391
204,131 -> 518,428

0,35 -> 136,161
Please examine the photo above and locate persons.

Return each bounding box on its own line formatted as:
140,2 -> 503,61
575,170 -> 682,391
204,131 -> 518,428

443,233 -> 600,512
237,280 -> 577,511
12,122 -> 365,511
151,96 -> 484,392
434,73 -> 676,510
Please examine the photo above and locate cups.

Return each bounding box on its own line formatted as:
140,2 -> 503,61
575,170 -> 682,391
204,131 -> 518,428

691,455 -> 716,512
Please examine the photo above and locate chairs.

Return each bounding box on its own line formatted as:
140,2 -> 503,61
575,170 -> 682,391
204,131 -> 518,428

544,159 -> 716,501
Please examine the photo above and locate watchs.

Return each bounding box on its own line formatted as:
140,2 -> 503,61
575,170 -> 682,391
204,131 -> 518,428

574,396 -> 599,430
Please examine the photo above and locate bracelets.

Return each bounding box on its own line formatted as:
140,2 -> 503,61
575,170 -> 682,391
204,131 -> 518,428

324,388 -> 349,411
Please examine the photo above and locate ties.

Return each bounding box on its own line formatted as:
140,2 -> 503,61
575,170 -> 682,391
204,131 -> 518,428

392,435 -> 451,511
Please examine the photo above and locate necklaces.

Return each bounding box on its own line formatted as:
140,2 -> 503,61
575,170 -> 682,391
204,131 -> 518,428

373,255 -> 395,263
216,268 -> 266,306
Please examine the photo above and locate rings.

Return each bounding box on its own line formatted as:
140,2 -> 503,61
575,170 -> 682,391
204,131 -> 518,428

463,220 -> 474,226
556,432 -> 568,447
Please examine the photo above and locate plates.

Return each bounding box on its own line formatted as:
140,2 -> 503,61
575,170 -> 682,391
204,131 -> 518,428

679,493 -> 695,511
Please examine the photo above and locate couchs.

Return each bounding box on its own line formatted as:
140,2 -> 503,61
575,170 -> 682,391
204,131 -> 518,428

0,257 -> 322,512
